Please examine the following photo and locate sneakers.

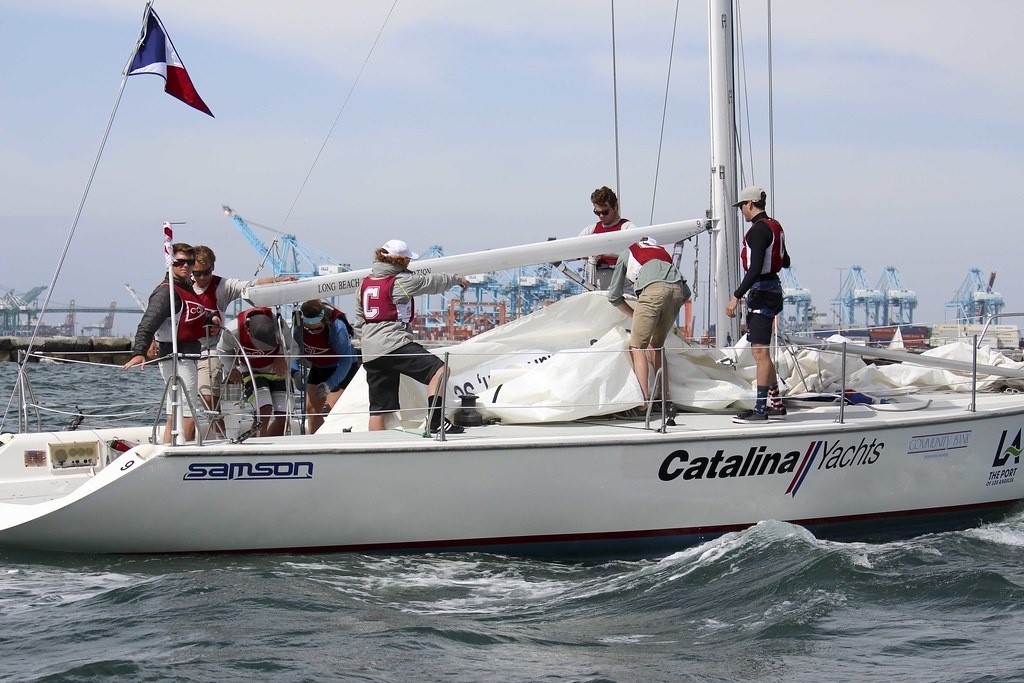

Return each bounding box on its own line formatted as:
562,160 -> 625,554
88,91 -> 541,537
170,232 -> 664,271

611,408 -> 651,421
732,409 -> 769,424
425,417 -> 464,434
768,406 -> 786,418
652,400 -> 673,413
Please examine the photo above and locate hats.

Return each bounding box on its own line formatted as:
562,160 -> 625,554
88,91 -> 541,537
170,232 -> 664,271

380,239 -> 419,260
248,315 -> 276,351
731,186 -> 766,207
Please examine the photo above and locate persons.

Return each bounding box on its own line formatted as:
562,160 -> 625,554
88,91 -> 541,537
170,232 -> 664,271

216,306 -> 299,437
725,186 -> 787,424
607,237 -> 688,420
145,245 -> 300,442
290,297 -> 360,434
562,185 -> 638,297
121,244 -> 222,444
354,239 -> 471,434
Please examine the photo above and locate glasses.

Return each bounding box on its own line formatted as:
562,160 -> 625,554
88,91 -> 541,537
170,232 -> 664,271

192,266 -> 212,276
304,323 -> 323,331
594,209 -> 615,215
740,203 -> 748,210
176,259 -> 195,266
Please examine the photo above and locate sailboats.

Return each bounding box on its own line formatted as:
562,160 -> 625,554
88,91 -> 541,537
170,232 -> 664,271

0,0 -> 1024,556
